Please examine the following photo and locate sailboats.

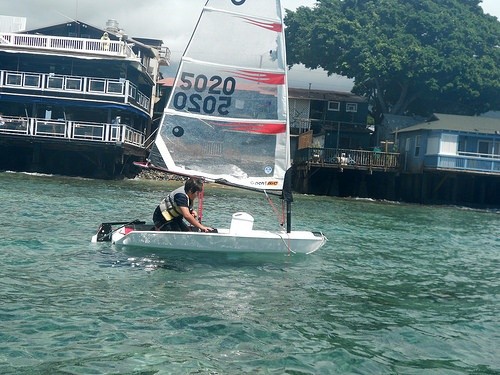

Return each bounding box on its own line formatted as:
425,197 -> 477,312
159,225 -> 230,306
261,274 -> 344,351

90,0 -> 328,254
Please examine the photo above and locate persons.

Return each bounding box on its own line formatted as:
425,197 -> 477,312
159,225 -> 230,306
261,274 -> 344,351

101,32 -> 111,52
152,178 -> 213,232
